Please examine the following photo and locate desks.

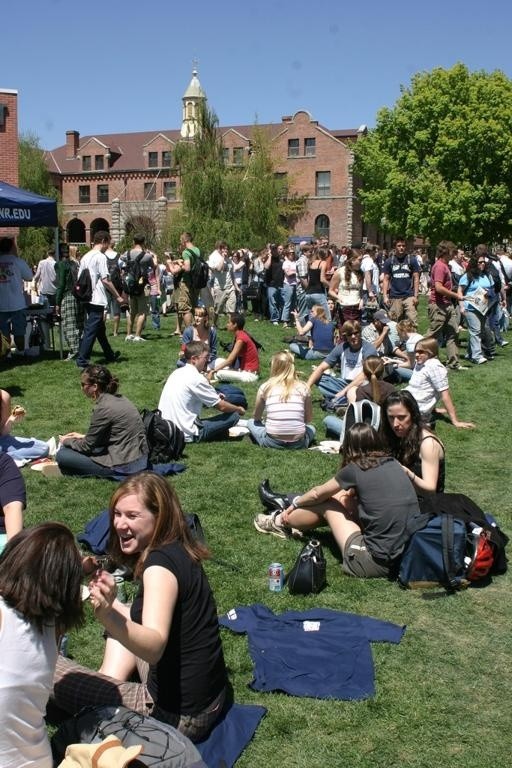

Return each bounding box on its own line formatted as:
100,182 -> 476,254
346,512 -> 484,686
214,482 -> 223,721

25,303 -> 54,354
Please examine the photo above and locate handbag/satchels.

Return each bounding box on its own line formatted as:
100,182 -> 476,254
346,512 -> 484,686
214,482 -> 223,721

288,541 -> 326,595
387,494 -> 508,590
51,705 -> 208,768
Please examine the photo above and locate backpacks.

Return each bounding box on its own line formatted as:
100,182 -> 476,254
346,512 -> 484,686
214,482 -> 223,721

344,400 -> 382,434
139,409 -> 185,464
105,252 -> 146,296
183,248 -> 209,289
71,251 -> 104,301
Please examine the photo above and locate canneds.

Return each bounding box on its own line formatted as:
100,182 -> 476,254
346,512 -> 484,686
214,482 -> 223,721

268,562 -> 283,592
114,576 -> 126,603
59,636 -> 68,658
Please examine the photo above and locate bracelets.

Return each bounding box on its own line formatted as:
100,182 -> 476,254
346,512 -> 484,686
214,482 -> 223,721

293,496 -> 300,508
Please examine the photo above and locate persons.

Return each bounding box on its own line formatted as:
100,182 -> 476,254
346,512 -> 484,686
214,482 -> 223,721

207,313 -> 259,383
0,232 -> 428,367
48,468 -> 228,744
0,520 -> 88,768
405,338 -> 475,428
307,320 -> 377,416
158,341 -> 246,443
56,365 -> 149,475
0,389 -> 49,458
427,241 -> 512,370
258,390 -> 446,510
0,451 -> 26,538
324,355 -> 395,435
254,423 -> 420,577
247,351 -> 316,449
177,308 -> 217,368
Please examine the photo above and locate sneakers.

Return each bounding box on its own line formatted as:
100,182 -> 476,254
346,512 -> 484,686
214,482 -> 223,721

43,465 -> 63,478
114,350 -> 119,361
254,478 -> 302,540
456,341 -> 509,370
125,336 -> 146,343
